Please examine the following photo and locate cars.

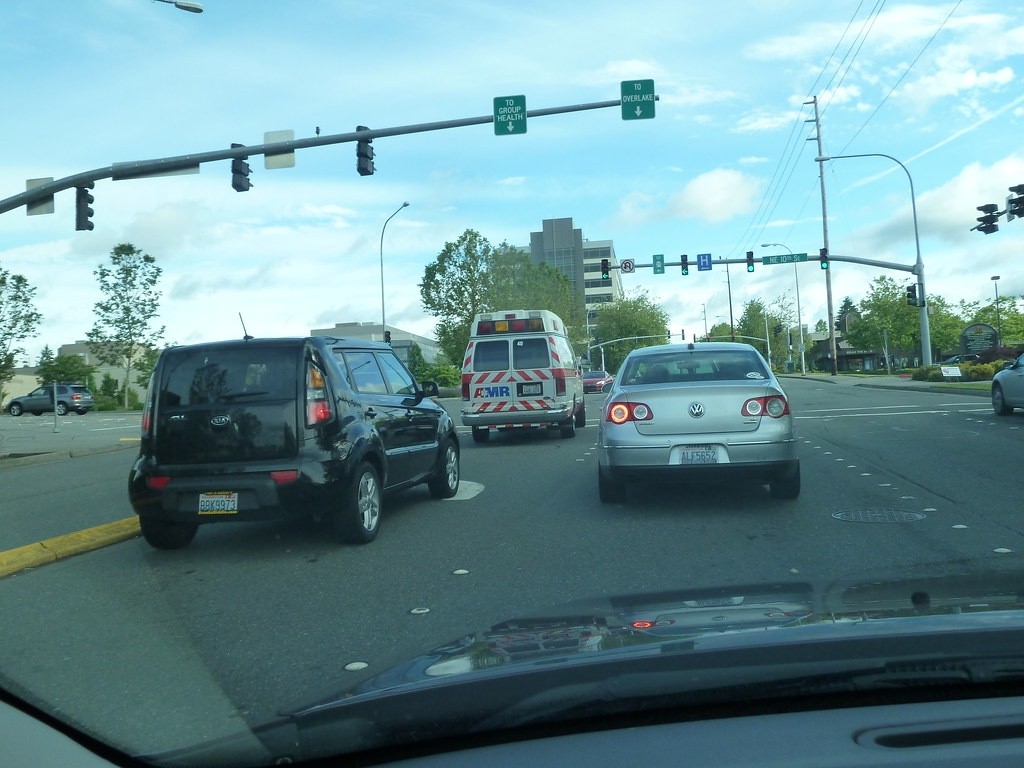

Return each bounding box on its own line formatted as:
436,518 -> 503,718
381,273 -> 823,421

992,351 -> 1024,417
581,371 -> 613,394
128,333 -> 460,543
933,354 -> 980,367
598,342 -> 802,508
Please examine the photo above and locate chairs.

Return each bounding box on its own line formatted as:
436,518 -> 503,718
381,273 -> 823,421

715,363 -> 742,381
644,366 -> 671,383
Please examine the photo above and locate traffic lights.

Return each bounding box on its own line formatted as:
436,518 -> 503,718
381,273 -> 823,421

681,255 -> 688,275
601,259 -> 608,279
907,286 -> 916,305
384,330 -> 391,346
76,182 -> 94,231
820,248 -> 828,269
746,252 -> 754,272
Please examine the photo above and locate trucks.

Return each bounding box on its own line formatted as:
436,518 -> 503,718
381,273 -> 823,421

461,308 -> 586,443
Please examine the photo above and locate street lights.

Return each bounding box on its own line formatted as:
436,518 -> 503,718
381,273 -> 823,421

814,154 -> 934,366
380,201 -> 410,341
762,243 -> 808,375
991,275 -> 1003,345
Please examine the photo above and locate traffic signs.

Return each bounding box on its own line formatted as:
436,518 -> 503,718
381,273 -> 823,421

493,95 -> 527,135
621,79 -> 655,120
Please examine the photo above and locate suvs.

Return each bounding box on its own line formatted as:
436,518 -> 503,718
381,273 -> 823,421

8,383 -> 96,416
